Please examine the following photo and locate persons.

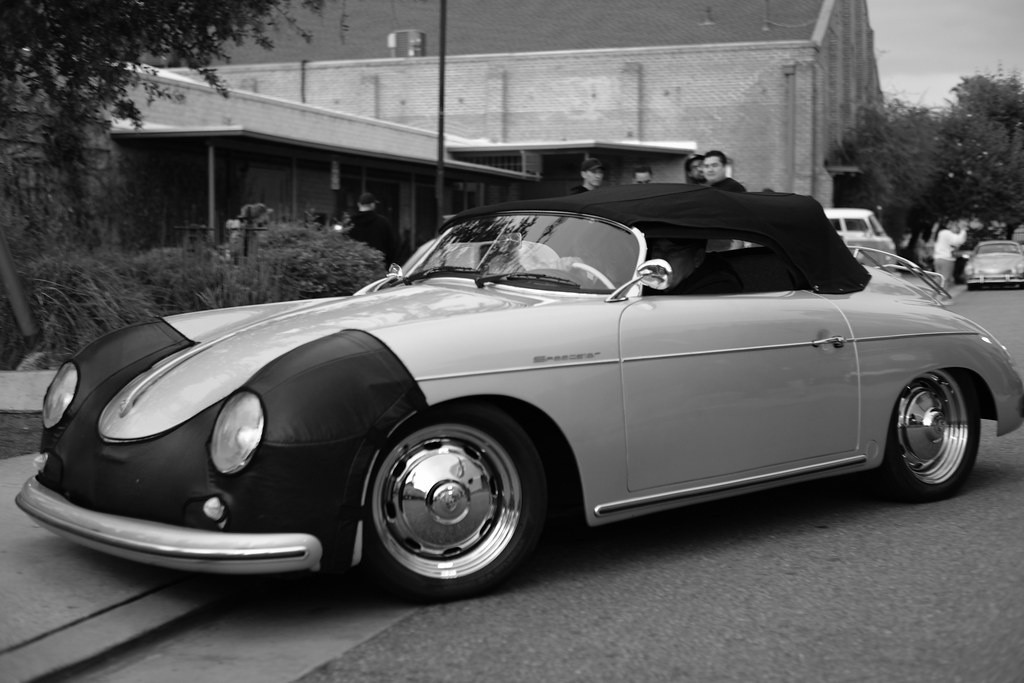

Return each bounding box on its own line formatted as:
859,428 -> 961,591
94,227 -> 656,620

569,158 -> 604,197
644,237 -> 742,296
631,164 -> 655,186
900,215 -> 1008,291
226,190 -> 399,272
684,149 -> 747,193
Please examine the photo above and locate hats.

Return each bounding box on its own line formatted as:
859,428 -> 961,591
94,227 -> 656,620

649,236 -> 707,251
685,154 -> 704,171
255,203 -> 275,217
237,204 -> 256,218
358,193 -> 381,205
581,158 -> 601,172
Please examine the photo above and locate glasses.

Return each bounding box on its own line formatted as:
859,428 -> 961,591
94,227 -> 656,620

239,217 -> 253,225
257,219 -> 267,227
343,215 -> 353,219
651,244 -> 694,258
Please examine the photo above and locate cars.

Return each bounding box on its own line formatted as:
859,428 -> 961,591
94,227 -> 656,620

14,181 -> 1024,601
963,240 -> 1024,290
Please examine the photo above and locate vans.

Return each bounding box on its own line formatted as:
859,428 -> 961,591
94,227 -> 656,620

739,207 -> 897,258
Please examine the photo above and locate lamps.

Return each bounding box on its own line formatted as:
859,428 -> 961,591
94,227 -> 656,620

331,160 -> 341,191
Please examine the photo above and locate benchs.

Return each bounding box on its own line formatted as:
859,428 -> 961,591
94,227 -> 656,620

707,246 -> 796,294
